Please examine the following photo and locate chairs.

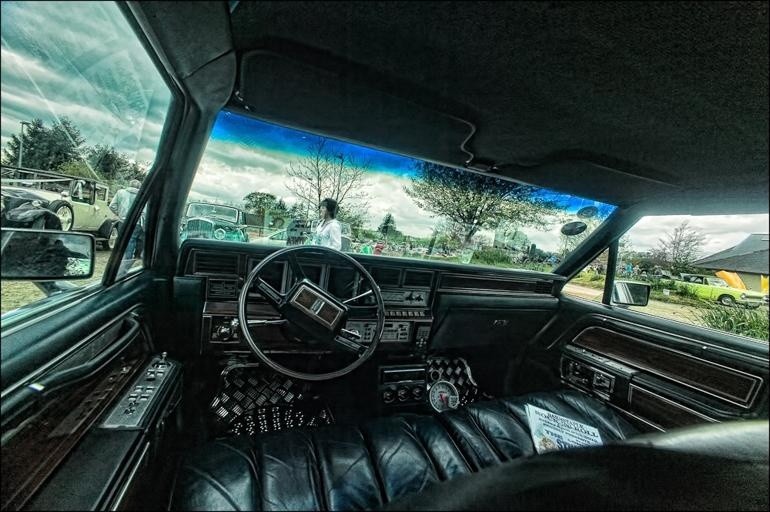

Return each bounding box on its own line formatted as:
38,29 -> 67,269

166,381 -> 765,511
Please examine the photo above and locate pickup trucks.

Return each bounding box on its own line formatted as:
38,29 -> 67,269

660,273 -> 768,308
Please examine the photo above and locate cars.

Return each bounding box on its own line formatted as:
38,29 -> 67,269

350,234 -> 456,257
1,190 -> 62,228
512,251 -> 668,285
251,228 -> 354,252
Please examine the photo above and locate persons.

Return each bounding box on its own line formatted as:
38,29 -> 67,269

312,198 -> 342,251
513,249 -> 641,276
108,179 -> 147,269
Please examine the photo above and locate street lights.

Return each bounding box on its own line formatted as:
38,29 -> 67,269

17,118 -> 32,167
336,152 -> 343,201
307,175 -> 314,218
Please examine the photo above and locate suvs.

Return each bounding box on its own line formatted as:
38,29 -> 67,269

178,203 -> 247,246
7,173 -> 122,252
